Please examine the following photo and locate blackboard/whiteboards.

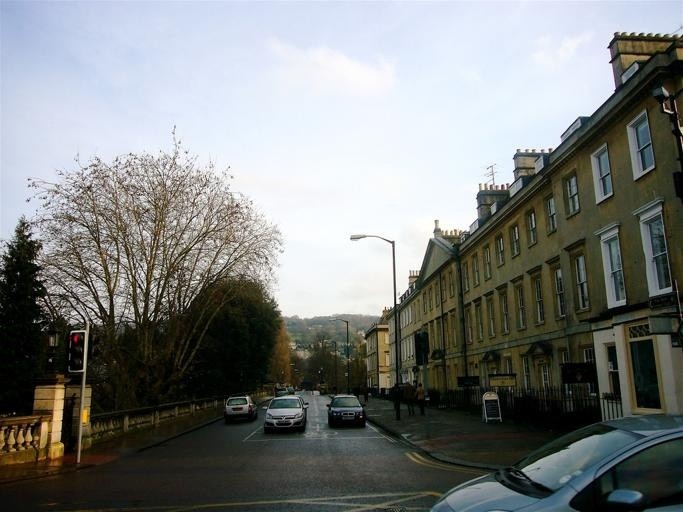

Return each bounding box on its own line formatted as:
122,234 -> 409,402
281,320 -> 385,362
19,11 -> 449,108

483,398 -> 503,420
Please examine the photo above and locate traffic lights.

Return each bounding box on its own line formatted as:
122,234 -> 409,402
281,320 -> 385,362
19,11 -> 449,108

68,330 -> 86,373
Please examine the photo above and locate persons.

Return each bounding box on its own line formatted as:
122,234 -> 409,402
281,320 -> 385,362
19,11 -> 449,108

403,382 -> 416,416
389,383 -> 401,407
414,382 -> 426,416
361,383 -> 369,401
287,385 -> 294,393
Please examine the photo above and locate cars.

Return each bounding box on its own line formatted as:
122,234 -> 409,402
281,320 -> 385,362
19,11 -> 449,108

326,396 -> 365,427
224,396 -> 257,421
262,387 -> 309,433
430,414 -> 683,512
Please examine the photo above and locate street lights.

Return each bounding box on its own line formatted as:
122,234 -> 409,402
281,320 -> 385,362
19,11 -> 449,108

329,318 -> 350,395
324,340 -> 337,396
351,234 -> 400,421
